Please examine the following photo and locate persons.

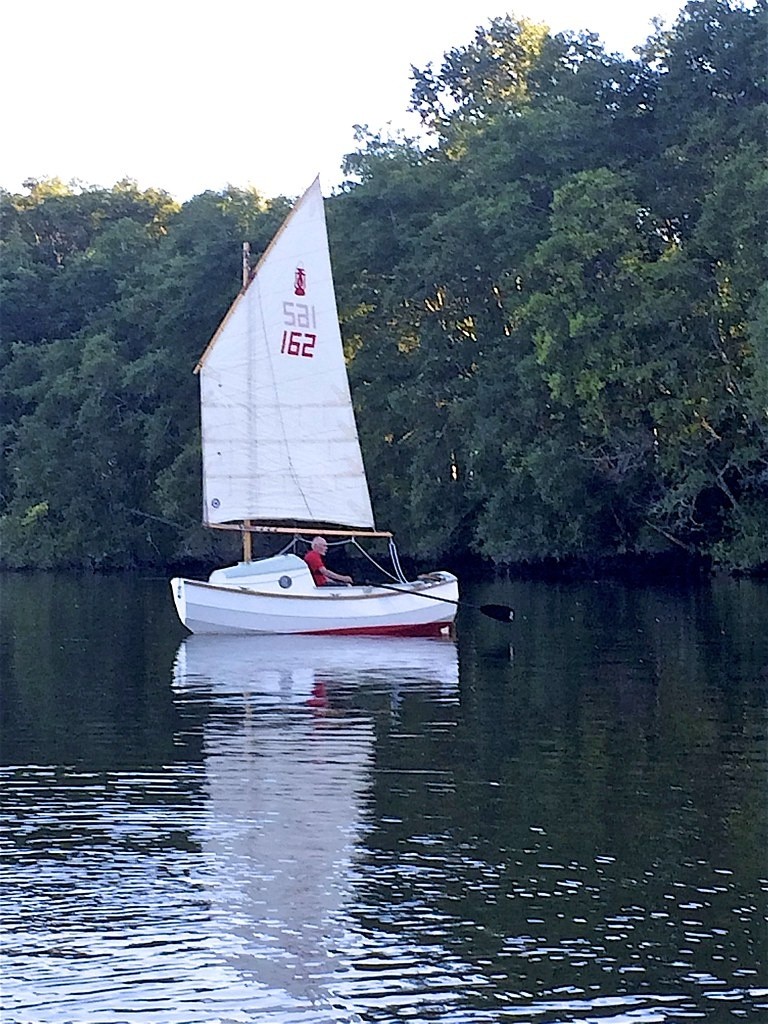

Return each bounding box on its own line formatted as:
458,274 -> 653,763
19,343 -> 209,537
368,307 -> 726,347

305,536 -> 353,587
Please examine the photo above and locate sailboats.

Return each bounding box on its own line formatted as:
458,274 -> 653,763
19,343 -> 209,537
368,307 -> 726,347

169,171 -> 461,638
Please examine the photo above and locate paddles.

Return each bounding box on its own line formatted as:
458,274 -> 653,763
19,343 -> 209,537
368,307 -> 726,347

353,578 -> 516,623
